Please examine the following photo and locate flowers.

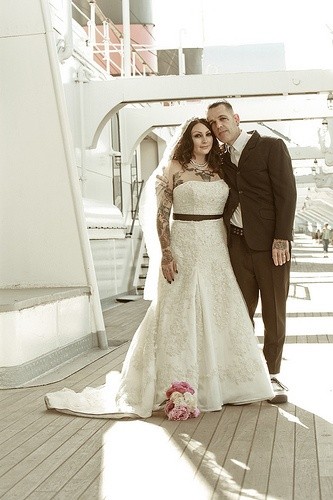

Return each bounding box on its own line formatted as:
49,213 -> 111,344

165,382 -> 200,422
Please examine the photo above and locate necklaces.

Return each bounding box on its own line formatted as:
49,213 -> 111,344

190,158 -> 209,171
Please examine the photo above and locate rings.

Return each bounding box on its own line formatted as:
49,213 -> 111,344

283,255 -> 286,257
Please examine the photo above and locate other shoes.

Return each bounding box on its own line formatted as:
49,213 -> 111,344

267,377 -> 288,404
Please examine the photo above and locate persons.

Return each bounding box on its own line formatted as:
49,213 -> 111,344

206,101 -> 297,401
321,224 -> 330,257
156,116 -> 234,413
